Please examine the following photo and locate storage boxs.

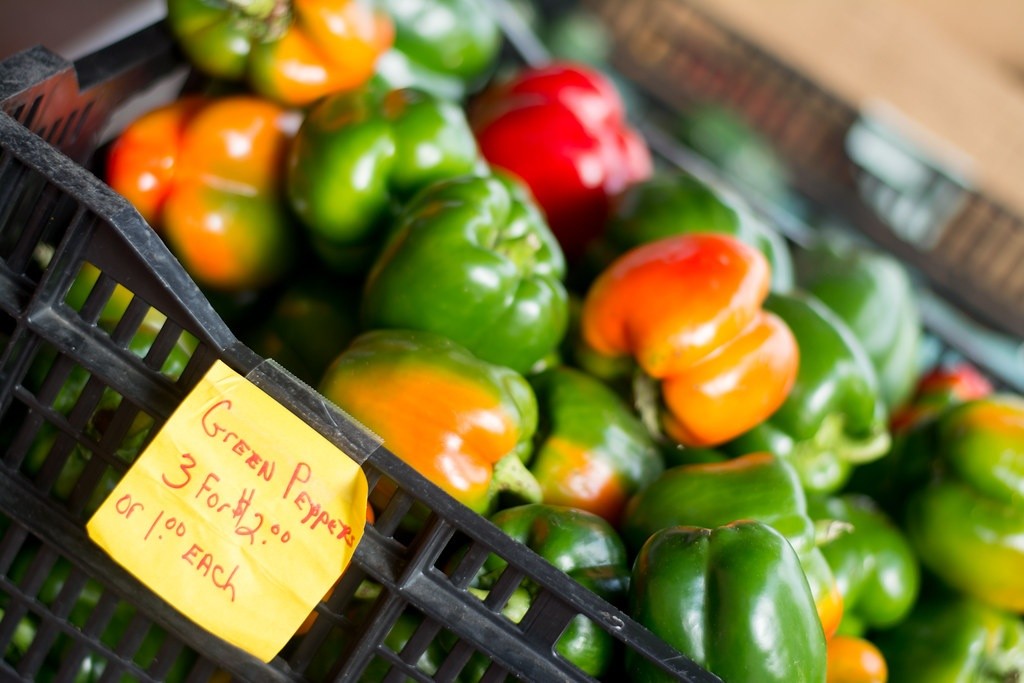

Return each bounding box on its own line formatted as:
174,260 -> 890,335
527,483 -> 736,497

0,0 -> 1024,683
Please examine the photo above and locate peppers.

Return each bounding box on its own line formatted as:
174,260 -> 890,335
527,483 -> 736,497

0,0 -> 1024,683
